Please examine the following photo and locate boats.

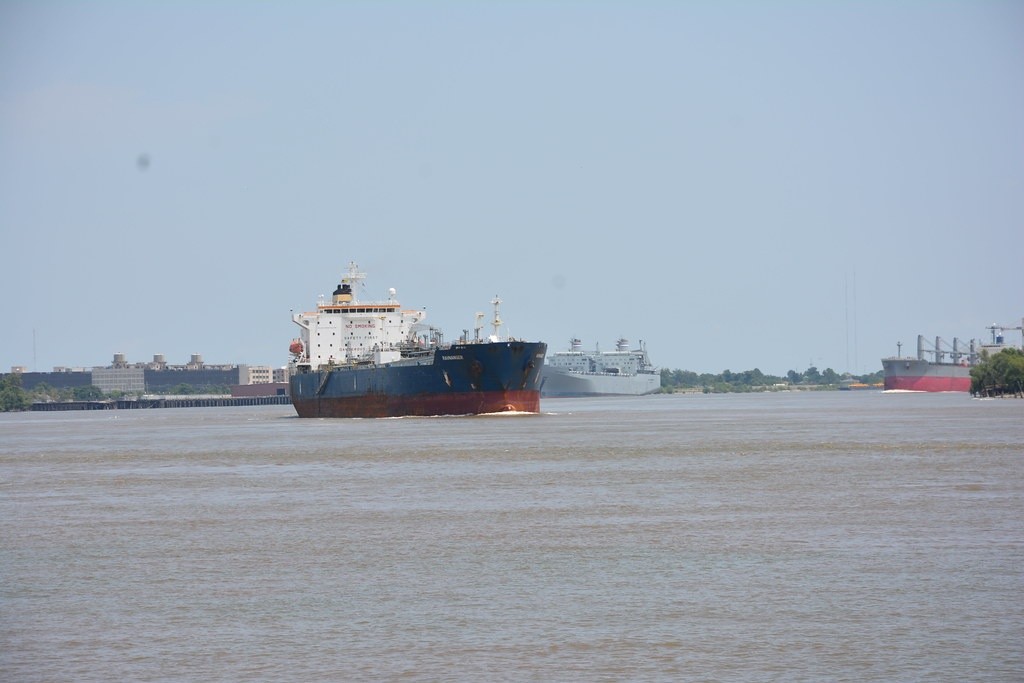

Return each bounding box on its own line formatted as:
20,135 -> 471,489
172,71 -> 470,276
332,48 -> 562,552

881,335 -> 978,392
288,261 -> 548,418
544,338 -> 660,396
